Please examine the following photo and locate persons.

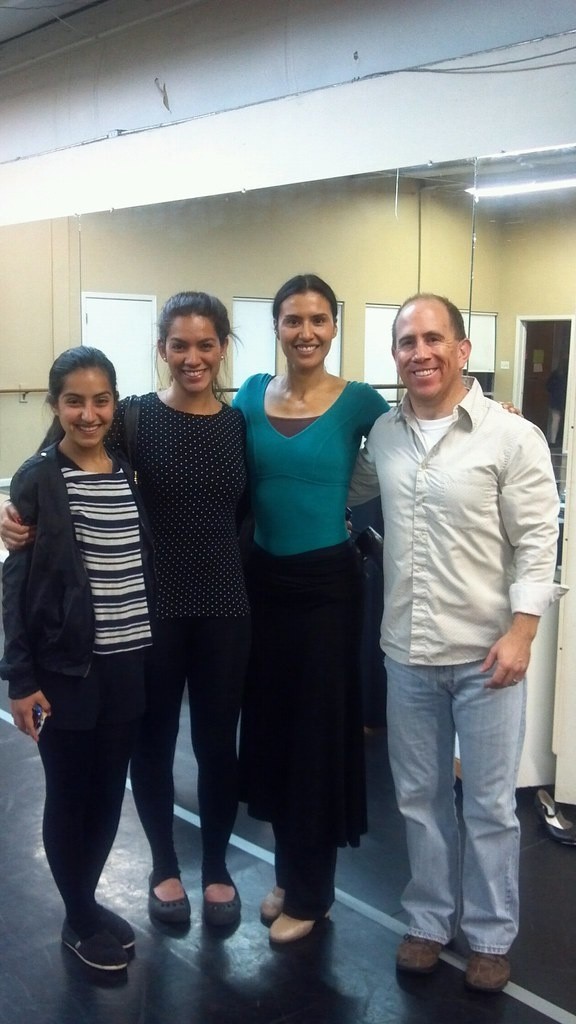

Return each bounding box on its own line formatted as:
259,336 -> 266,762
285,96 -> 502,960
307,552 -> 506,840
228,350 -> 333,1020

1,295 -> 251,927
0,342 -> 154,974
347,294 -> 561,999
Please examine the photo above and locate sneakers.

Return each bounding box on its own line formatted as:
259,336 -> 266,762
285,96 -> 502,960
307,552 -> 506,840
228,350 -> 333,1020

465,945 -> 510,993
397,931 -> 442,974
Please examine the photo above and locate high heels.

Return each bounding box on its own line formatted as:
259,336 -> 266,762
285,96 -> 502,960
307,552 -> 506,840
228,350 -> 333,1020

534,788 -> 576,847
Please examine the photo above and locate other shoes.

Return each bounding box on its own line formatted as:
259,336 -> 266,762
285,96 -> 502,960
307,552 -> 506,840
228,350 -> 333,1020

62,917 -> 128,970
201,873 -> 242,926
269,907 -> 331,942
364,714 -> 388,734
261,881 -> 284,922
94,904 -> 137,948
148,868 -> 191,924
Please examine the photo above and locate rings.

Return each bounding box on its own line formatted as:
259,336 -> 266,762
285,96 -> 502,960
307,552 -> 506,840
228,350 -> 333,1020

511,678 -> 520,684
230,275 -> 526,949
